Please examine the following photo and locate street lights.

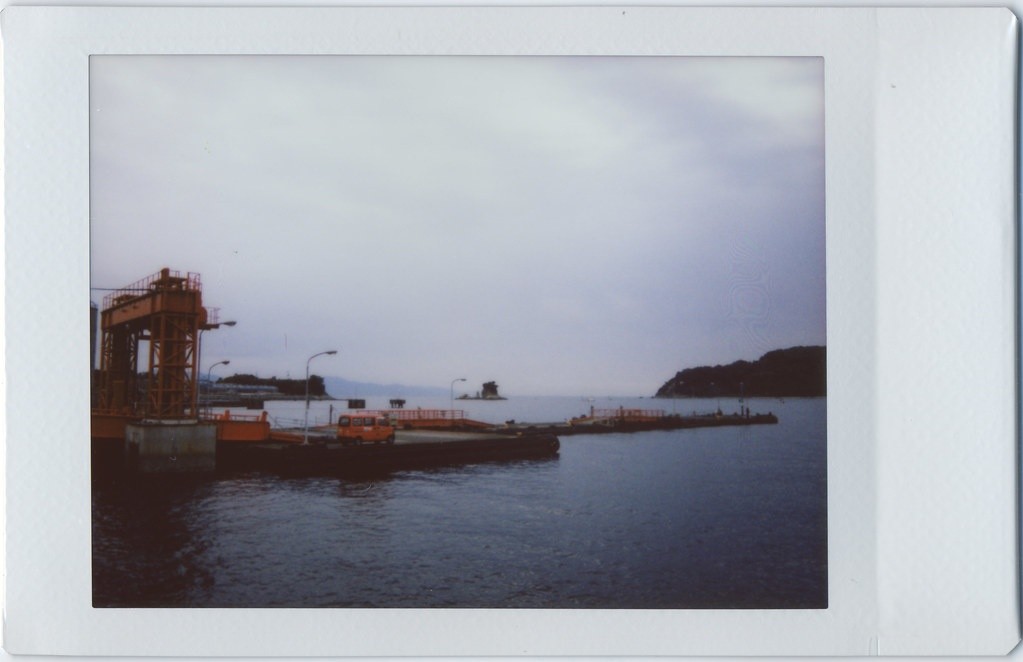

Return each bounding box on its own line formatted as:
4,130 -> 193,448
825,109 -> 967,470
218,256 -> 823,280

304,350 -> 338,445
196,320 -> 237,415
206,361 -> 230,418
450,379 -> 467,420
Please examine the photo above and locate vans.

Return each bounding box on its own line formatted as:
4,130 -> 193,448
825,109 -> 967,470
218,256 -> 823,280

337,414 -> 395,445
